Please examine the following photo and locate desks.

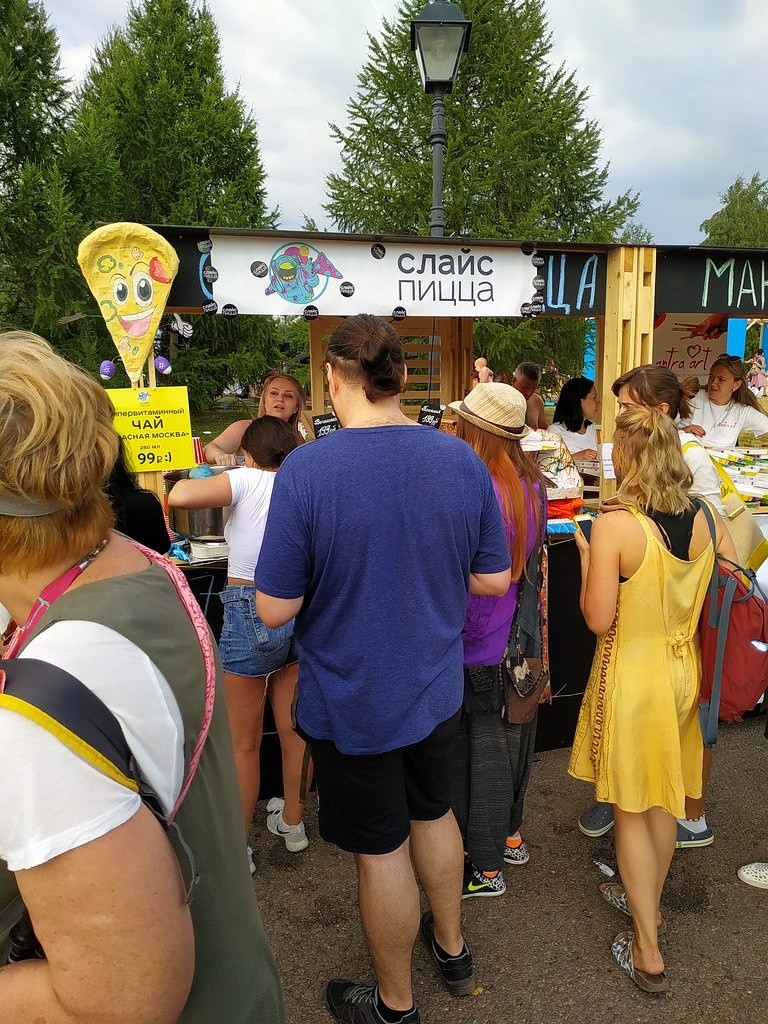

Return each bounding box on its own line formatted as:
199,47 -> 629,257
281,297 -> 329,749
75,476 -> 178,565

166,455 -> 768,802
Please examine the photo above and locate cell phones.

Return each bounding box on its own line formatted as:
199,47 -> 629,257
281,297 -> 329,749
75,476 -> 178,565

573,514 -> 593,545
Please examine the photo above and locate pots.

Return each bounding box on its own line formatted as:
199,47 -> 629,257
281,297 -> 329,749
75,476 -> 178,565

163,466 -> 247,540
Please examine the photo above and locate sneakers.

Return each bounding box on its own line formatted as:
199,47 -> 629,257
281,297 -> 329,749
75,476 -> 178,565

326,978 -> 421,1024
421,911 -> 477,996
460,862 -> 506,899
267,797 -> 309,851
501,830 -> 531,866
248,845 -> 256,874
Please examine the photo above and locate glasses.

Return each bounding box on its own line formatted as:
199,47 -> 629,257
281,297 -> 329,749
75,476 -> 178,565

717,353 -> 743,364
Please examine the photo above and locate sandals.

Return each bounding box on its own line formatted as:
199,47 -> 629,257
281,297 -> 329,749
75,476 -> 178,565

674,818 -> 715,849
577,801 -> 614,838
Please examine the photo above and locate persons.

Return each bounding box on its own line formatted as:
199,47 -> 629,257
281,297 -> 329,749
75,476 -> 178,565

0,330 -> 297,1024
91,349 -> 768,899
253,313 -> 513,1024
564,405 -> 743,992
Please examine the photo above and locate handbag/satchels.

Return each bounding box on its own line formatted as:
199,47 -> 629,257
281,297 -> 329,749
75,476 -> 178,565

502,656 -> 549,725
722,490 -> 768,588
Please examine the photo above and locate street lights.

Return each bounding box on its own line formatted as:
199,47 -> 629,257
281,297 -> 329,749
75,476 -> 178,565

409,0 -> 473,238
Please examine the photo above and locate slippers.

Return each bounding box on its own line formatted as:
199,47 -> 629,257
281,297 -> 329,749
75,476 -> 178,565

597,883 -> 667,937
611,931 -> 668,993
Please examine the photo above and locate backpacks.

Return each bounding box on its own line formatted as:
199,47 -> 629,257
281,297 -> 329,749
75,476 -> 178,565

695,498 -> 768,748
750,356 -> 762,375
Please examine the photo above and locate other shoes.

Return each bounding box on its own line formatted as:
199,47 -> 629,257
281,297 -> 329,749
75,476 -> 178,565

738,862 -> 768,890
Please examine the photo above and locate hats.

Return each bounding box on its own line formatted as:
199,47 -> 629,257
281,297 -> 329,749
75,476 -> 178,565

448,382 -> 529,440
